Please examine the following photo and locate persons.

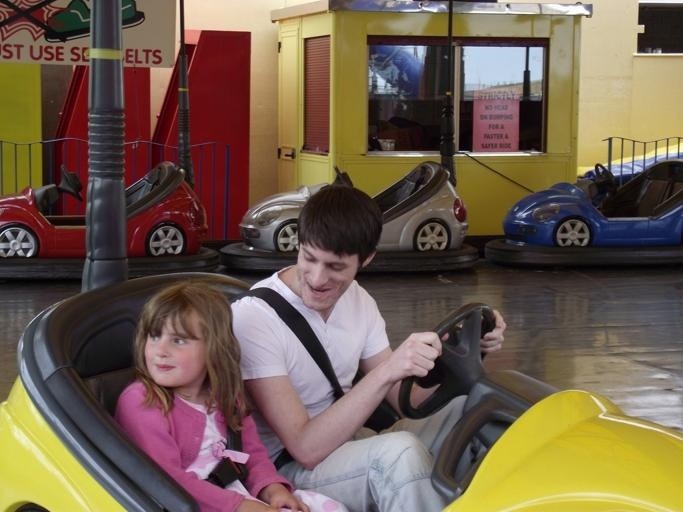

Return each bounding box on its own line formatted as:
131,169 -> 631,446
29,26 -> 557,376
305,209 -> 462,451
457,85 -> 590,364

230,185 -> 507,512
114,282 -> 347,512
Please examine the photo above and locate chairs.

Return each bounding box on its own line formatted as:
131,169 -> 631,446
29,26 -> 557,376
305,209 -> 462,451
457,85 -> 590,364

33,275 -> 401,511
372,160 -> 445,219
607,158 -> 683,217
124,160 -> 177,216
